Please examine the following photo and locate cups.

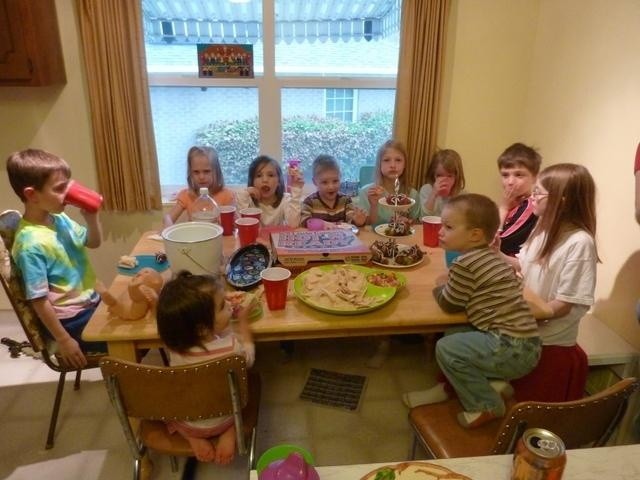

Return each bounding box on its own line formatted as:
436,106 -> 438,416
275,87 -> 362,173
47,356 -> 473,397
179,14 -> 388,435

438,179 -> 451,196
306,217 -> 325,231
259,267 -> 292,310
62,181 -> 105,215
421,216 -> 442,247
218,205 -> 262,248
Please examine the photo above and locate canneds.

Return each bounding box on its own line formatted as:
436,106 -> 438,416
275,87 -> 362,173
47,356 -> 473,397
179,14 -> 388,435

512,428 -> 567,480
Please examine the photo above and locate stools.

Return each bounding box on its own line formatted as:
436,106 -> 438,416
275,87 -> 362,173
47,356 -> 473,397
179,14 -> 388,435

572,313 -> 640,396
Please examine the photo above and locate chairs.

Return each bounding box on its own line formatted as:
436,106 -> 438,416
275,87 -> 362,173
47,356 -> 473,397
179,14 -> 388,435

0,207 -> 149,453
99,352 -> 262,478
404,377 -> 638,460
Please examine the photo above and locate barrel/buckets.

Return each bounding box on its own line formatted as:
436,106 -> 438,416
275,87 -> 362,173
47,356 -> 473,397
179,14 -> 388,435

161,220 -> 225,287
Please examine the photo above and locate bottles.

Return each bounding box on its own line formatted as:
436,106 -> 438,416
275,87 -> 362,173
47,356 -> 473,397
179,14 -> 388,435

189,187 -> 220,225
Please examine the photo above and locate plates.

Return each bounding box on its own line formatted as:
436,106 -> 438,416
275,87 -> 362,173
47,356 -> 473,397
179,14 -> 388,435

369,246 -> 424,268
378,197 -> 416,211
293,264 -> 408,315
375,224 -> 416,239
445,249 -> 462,269
224,243 -> 272,289
116,255 -> 170,275
228,303 -> 263,322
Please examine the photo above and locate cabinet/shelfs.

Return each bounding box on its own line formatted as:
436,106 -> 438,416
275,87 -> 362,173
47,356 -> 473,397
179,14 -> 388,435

0,0 -> 67,90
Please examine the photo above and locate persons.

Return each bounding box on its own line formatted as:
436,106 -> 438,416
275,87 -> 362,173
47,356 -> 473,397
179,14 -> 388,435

399,162 -> 598,409
633,141 -> 640,226
155,144 -> 234,236
416,149 -> 467,224
234,155 -> 306,228
492,142 -> 543,257
298,153 -> 370,228
431,192 -> 543,427
153,267 -> 257,466
7,147 -> 151,367
359,138 -> 421,225
